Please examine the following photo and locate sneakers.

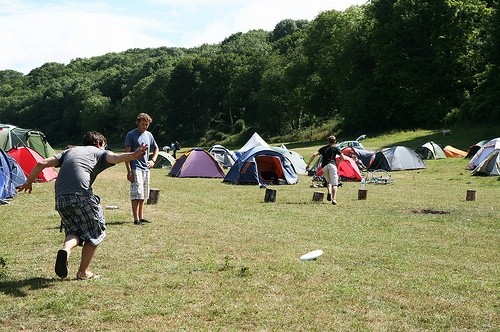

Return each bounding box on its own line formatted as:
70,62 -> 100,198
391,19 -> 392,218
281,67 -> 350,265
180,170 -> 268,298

134,219 -> 151,226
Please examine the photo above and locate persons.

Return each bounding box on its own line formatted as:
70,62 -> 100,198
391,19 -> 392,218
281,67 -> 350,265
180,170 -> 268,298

123,113 -> 159,226
161,140 -> 182,160
15,130 -> 148,280
305,136 -> 343,205
260,163 -> 279,185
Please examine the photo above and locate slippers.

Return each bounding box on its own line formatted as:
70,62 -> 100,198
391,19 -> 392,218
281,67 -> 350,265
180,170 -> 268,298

77,272 -> 103,280
55,249 -> 69,278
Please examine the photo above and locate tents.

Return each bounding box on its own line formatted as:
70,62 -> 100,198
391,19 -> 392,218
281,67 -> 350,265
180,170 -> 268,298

168,147 -> 226,179
470,148 -> 500,177
5,146 -> 59,183
238,132 -> 271,152
221,145 -> 299,189
366,145 -> 428,172
0,123 -> 60,160
280,143 -> 312,174
304,134 -> 378,177
442,144 -> 468,159
463,139 -> 488,159
310,153 -> 365,182
0,147 -> 29,205
148,151 -> 176,169
207,144 -> 238,168
412,140 -> 447,160
464,137 -> 500,170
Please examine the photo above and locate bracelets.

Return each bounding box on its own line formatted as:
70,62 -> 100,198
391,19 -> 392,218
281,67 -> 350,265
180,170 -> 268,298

151,159 -> 156,164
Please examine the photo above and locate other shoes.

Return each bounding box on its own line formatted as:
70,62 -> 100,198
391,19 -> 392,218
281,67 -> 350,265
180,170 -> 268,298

327,193 -> 337,205
